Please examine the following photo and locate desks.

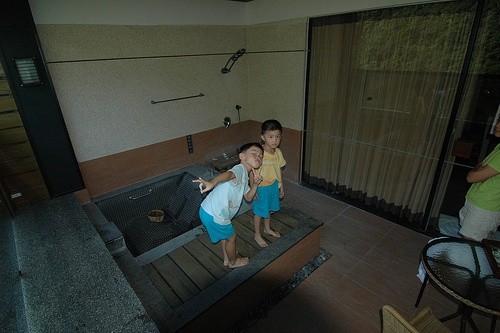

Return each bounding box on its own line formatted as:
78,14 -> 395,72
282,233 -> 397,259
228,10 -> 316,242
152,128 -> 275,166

415,237 -> 500,333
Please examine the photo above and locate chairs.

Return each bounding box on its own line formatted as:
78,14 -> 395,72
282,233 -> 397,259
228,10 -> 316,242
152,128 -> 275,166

382,305 -> 452,333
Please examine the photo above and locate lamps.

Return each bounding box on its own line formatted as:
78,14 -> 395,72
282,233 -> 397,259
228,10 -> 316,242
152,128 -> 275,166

12,57 -> 44,88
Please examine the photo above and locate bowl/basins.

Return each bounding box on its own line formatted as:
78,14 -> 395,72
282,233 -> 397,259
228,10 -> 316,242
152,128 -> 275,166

147,210 -> 164,223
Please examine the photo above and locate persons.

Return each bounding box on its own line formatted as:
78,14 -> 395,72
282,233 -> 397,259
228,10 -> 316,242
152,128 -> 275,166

458,122 -> 500,242
191,142 -> 264,269
249,119 -> 286,248
